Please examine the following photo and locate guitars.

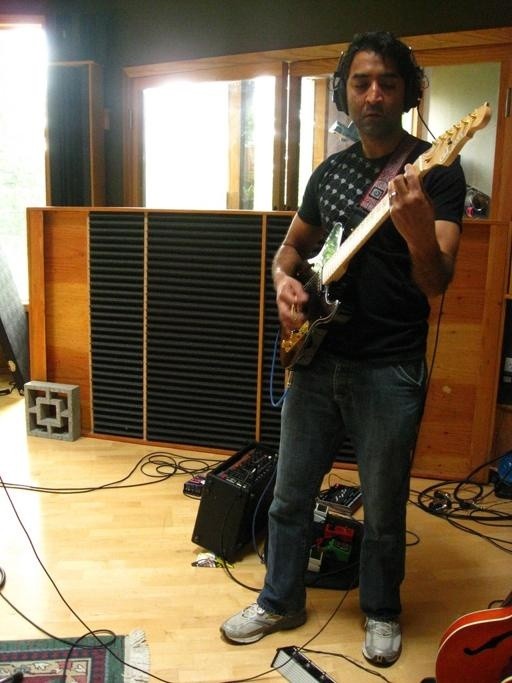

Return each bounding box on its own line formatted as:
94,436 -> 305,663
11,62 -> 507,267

435,608 -> 511,683
279,100 -> 494,371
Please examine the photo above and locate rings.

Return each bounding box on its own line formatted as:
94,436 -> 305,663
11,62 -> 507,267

388,191 -> 397,201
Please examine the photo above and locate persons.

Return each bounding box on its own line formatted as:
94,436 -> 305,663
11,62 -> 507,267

218,27 -> 466,670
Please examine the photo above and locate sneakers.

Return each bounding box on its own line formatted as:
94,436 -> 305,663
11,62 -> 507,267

362,614 -> 402,665
219,602 -> 308,644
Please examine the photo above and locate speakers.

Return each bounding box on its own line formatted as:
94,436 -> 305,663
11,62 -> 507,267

192,442 -> 282,565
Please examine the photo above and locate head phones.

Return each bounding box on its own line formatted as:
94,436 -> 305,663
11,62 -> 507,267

330,33 -> 424,113
429,489 -> 481,514
